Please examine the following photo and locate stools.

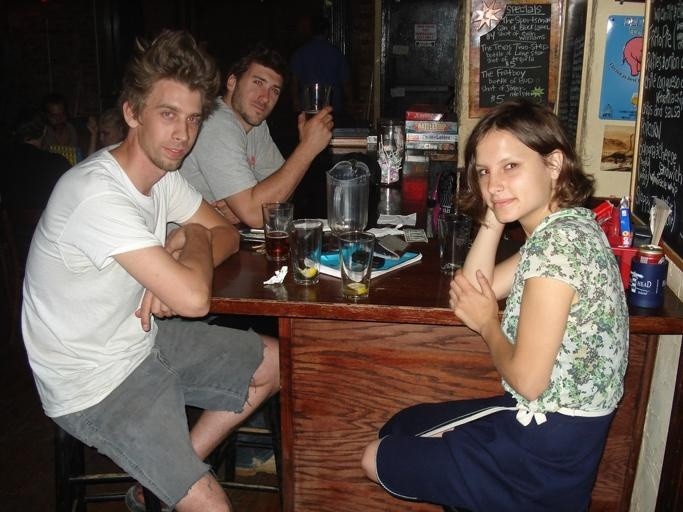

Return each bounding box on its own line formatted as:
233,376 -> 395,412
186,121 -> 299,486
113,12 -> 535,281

209,317 -> 284,509
52,419 -> 163,512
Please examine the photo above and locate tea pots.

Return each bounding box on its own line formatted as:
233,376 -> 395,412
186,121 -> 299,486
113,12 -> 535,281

327,160 -> 372,238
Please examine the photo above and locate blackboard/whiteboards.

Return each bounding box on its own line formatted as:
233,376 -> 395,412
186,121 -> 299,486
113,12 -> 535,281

470,3 -> 561,120
626,0 -> 683,275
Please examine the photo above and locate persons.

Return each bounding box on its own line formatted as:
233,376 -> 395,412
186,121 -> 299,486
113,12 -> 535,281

360,101 -> 631,508
86,107 -> 128,158
177,52 -> 335,229
39,94 -> 88,179
327,126 -> 371,156
1,105 -> 72,211
404,104 -> 459,163
20,31 -> 280,512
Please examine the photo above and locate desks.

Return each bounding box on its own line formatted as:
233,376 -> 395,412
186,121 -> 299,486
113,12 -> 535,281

211,184 -> 682,511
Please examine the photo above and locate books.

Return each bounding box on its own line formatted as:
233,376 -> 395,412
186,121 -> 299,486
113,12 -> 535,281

303,251 -> 423,284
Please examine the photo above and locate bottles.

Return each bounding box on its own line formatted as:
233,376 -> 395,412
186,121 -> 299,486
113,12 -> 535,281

635,243 -> 665,265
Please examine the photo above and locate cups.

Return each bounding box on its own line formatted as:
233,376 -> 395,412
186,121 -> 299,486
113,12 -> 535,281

261,202 -> 294,262
435,212 -> 468,276
625,257 -> 669,309
287,218 -> 323,286
338,229 -> 374,302
304,84 -> 330,116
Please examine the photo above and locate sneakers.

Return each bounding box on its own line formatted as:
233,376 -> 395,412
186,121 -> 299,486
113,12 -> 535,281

124,484 -> 174,512
